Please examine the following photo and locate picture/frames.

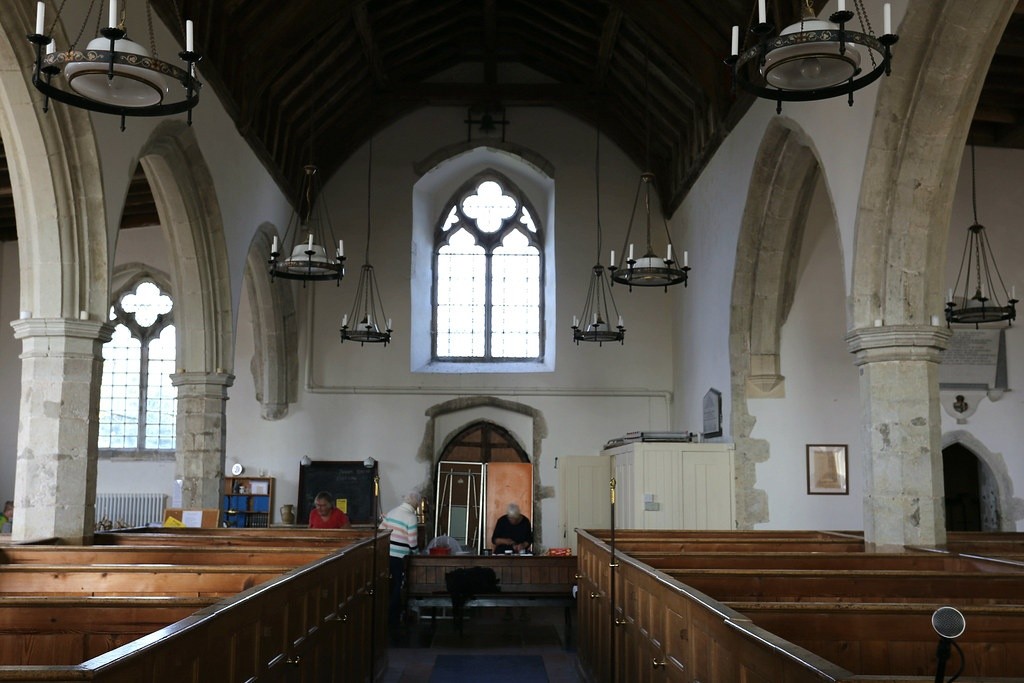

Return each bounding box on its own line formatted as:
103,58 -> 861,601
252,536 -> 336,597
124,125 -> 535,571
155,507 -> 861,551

806,444 -> 849,495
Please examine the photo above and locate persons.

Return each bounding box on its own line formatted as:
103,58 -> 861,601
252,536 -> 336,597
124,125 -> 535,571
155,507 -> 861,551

492,504 -> 532,554
0,501 -> 12,534
376,492 -> 422,642
308,492 -> 352,529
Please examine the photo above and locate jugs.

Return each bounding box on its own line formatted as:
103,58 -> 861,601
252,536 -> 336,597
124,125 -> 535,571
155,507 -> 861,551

280,503 -> 296,524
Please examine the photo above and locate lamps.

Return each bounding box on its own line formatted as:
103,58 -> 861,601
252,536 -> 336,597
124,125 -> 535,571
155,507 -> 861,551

724,0 -> 899,115
607,109 -> 693,294
946,147 -> 1019,330
572,113 -> 626,347
25,0 -> 203,133
301,455 -> 311,466
268,57 -> 348,288
340,100 -> 394,347
364,457 -> 374,468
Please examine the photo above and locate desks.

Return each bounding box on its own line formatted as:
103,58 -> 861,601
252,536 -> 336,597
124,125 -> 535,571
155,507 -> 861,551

574,526 -> 1024,683
0,527 -> 392,683
404,553 -> 578,592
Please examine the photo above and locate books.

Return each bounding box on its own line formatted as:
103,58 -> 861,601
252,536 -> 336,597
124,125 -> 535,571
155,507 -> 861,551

245,513 -> 268,527
248,496 -> 254,513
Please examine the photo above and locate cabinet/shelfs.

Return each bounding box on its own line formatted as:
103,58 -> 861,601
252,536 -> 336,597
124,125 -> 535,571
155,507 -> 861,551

223,477 -> 275,528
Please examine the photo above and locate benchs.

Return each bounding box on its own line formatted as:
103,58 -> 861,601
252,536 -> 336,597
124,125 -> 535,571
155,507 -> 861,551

405,588 -> 577,655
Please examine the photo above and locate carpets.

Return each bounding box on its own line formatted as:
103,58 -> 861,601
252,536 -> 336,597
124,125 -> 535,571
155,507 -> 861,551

427,655 -> 550,683
